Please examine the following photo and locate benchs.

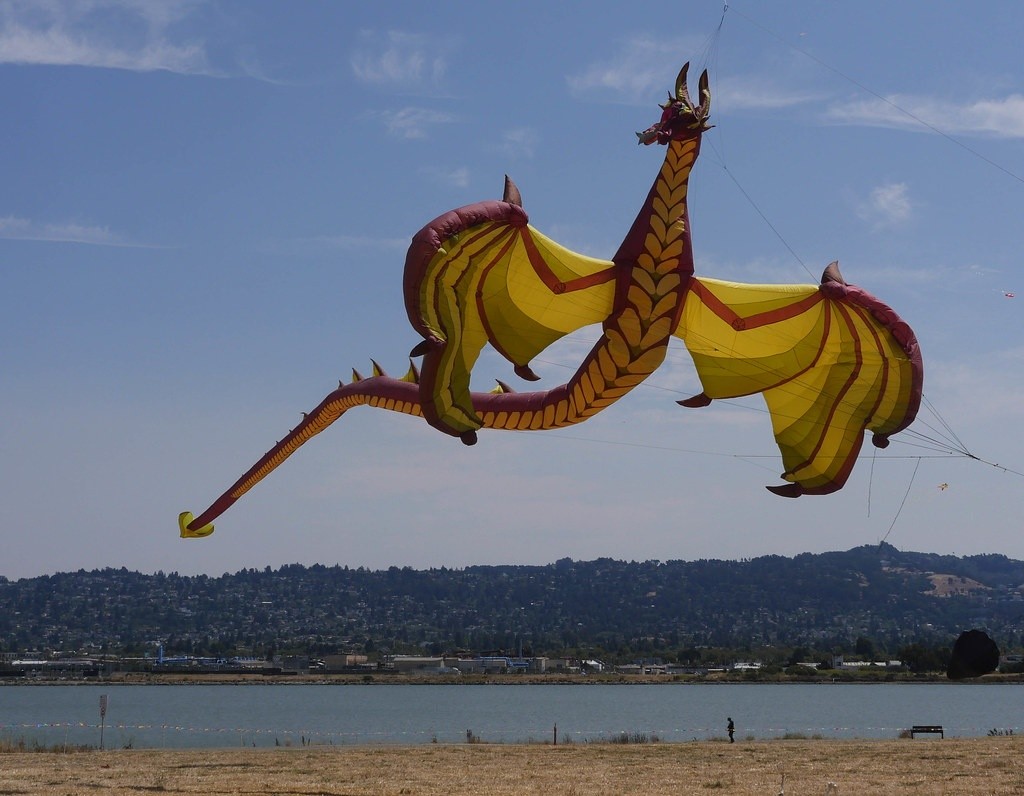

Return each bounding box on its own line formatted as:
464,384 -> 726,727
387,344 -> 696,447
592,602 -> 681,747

910,725 -> 944,739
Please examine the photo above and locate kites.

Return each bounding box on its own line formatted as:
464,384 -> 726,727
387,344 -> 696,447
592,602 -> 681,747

179,62 -> 924,539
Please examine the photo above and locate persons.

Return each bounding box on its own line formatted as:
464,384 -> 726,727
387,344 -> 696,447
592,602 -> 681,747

726,717 -> 735,743
